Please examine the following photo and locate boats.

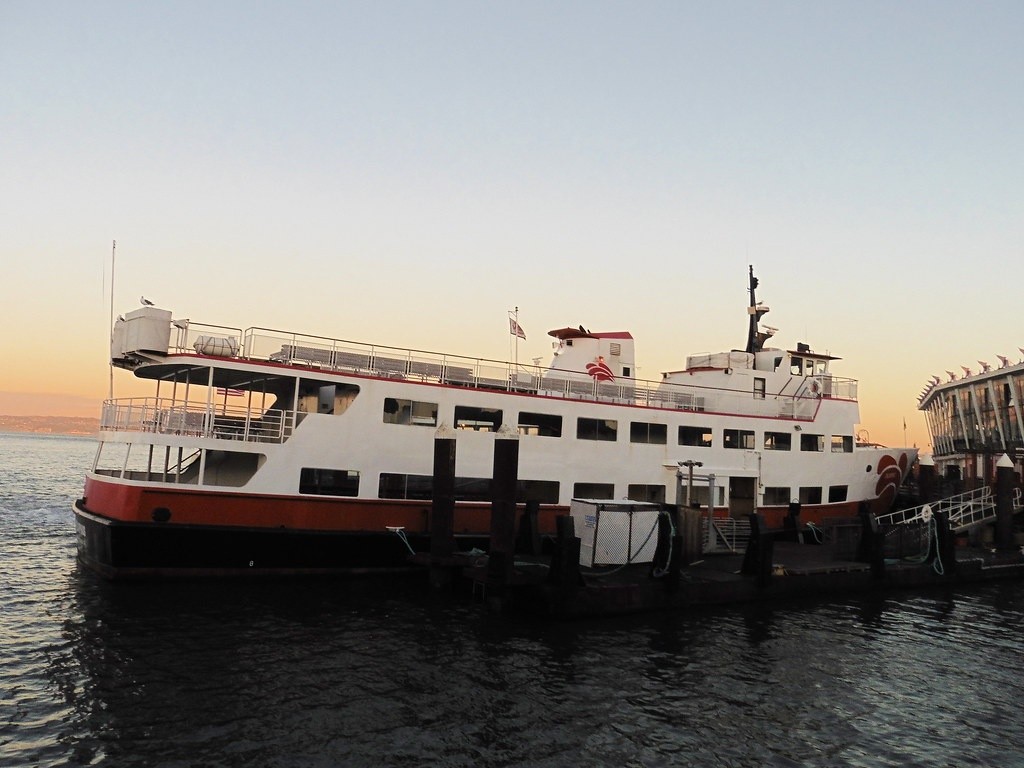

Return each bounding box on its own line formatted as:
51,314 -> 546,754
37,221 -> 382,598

914,350 -> 1024,581
72,240 -> 922,603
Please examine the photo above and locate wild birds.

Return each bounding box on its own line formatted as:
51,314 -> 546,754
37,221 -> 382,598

140,295 -> 155,307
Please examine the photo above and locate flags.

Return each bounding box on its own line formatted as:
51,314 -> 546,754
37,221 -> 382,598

509,318 -> 527,341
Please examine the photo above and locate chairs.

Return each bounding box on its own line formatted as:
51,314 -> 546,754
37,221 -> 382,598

143,406 -> 266,443
268,344 -> 693,411
